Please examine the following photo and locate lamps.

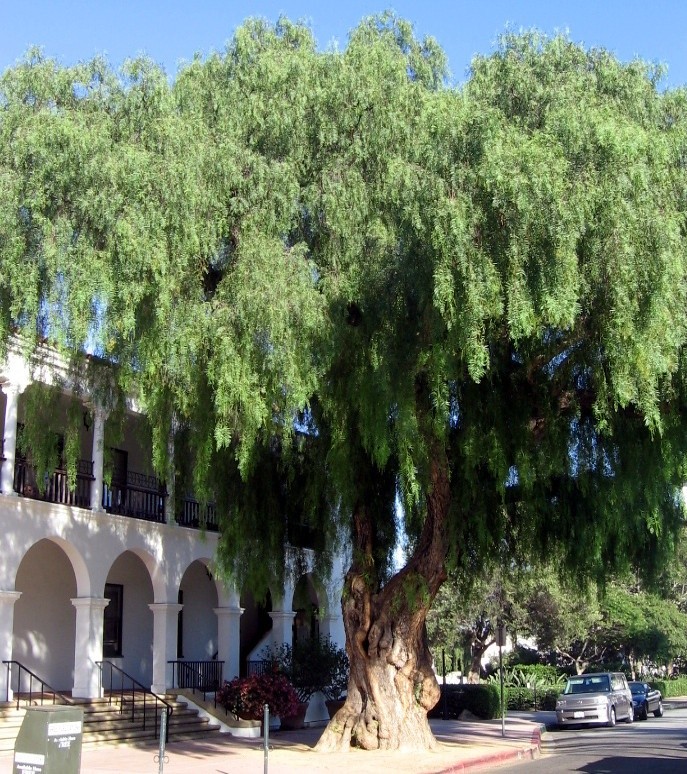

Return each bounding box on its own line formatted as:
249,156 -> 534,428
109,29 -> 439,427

83,410 -> 92,430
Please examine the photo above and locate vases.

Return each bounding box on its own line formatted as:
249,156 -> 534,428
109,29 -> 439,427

241,711 -> 253,721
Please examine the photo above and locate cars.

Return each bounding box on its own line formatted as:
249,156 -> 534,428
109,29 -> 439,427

626,680 -> 664,720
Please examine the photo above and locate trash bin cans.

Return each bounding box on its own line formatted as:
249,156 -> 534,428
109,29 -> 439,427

13,706 -> 84,774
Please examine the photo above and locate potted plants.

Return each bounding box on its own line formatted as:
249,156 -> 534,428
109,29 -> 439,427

255,634 -> 349,731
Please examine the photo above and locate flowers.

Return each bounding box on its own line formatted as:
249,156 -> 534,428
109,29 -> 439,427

216,671 -> 301,717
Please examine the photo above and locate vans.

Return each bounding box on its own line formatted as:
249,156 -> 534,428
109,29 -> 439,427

555,672 -> 635,730
